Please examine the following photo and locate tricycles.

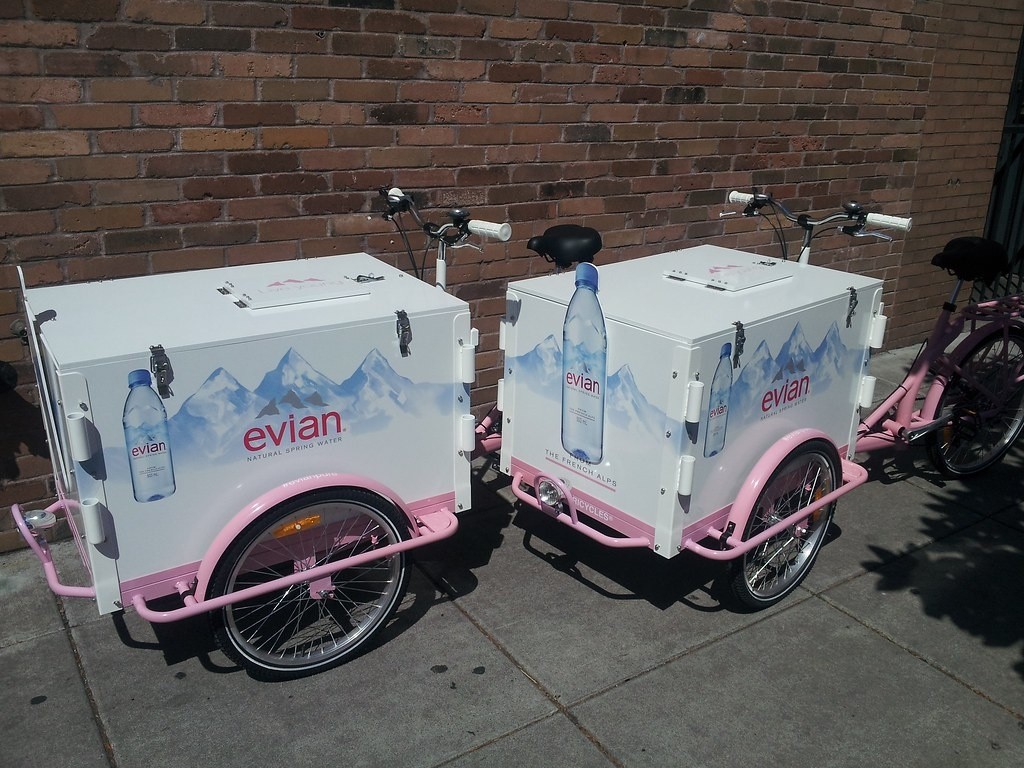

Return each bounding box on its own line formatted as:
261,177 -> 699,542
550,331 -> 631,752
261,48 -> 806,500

11,183 -> 512,682
499,186 -> 1024,614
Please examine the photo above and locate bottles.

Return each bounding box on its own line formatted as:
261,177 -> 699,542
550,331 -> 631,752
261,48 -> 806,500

559,261 -> 607,464
704,343 -> 733,458
123,369 -> 177,502
